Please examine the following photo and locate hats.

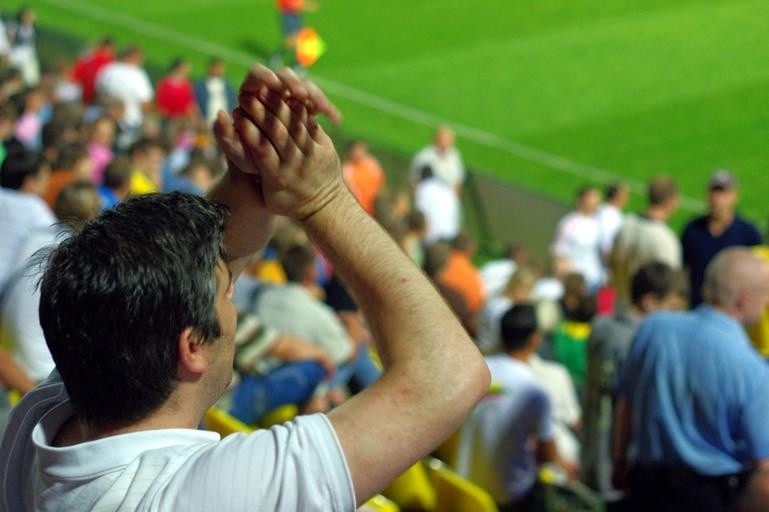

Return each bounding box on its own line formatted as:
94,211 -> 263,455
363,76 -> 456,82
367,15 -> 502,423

709,168 -> 734,191
